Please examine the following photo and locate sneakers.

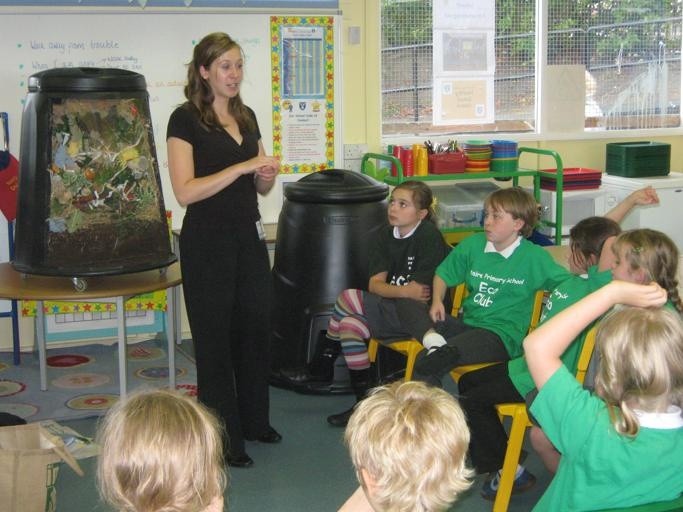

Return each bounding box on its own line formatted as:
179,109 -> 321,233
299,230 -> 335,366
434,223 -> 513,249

482,464 -> 536,500
416,344 -> 458,375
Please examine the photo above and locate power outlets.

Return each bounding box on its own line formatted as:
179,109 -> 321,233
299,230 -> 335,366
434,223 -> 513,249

344,144 -> 353,160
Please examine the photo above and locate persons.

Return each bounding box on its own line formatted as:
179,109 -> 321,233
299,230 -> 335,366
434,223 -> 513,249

396,186 -> 573,389
166,30 -> 284,470
520,279 -> 683,511
457,184 -> 660,502
278,179 -> 452,428
337,380 -> 492,512
93,382 -> 230,512
529,226 -> 683,477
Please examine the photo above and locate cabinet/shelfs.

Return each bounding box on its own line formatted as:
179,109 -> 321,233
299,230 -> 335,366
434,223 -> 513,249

360,153 -> 563,248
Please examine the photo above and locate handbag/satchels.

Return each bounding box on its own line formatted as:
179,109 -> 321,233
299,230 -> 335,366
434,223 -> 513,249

0,420 -> 83,511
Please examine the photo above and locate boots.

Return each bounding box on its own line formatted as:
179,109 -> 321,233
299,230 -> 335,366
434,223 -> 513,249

327,373 -> 378,428
284,329 -> 340,388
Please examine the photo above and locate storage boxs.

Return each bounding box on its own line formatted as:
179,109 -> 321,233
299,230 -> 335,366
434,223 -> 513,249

428,182 -> 484,230
456,179 -> 503,208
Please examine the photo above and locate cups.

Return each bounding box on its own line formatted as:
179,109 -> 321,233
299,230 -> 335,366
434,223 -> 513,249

392,144 -> 428,177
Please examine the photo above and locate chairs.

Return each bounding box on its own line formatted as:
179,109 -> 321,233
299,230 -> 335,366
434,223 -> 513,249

363,284 -> 464,390
488,322 -> 598,512
444,286 -> 543,388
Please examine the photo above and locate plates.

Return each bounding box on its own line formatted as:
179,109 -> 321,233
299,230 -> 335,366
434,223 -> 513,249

490,140 -> 518,181
466,138 -> 491,171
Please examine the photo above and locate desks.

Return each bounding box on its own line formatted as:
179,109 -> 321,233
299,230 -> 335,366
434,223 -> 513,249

172,219 -> 278,346
0,258 -> 183,402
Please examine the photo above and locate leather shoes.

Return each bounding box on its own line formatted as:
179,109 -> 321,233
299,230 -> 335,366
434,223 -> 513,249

256,422 -> 281,443
227,451 -> 252,466
